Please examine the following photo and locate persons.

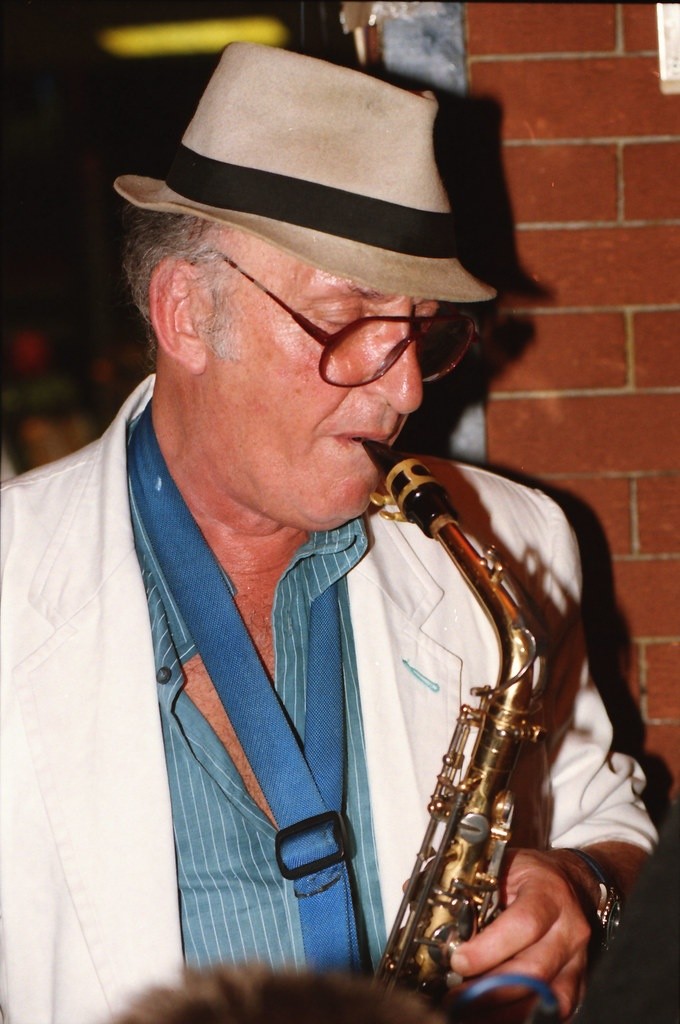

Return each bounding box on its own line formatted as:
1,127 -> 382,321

0,40 -> 662,1023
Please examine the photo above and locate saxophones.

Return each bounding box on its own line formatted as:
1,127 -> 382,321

357,435 -> 569,1014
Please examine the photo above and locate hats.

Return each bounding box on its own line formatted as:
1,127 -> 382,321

113,42 -> 496,302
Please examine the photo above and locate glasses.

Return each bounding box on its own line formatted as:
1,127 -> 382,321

187,250 -> 475,388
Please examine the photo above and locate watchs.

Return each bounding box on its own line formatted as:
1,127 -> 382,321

549,846 -> 622,966
